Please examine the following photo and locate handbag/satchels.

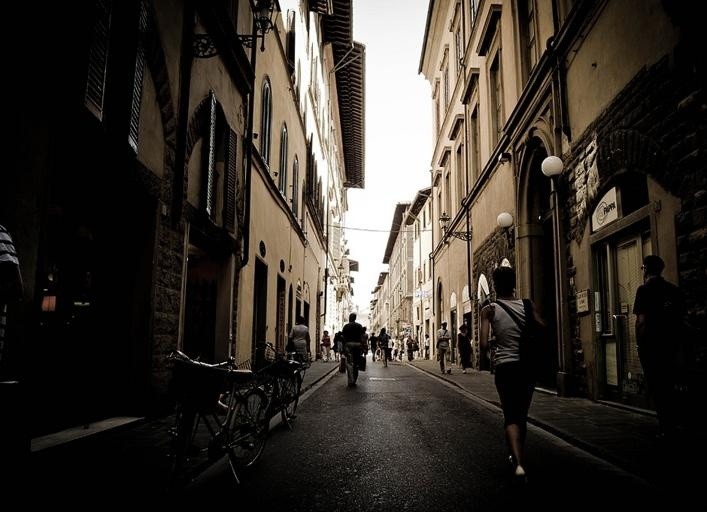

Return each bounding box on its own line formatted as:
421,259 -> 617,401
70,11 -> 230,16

339,354 -> 346,373
286,339 -> 294,352
358,355 -> 366,371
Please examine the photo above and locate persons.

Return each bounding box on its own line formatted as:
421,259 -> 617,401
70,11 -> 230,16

0,224 -> 25,369
633,255 -> 689,439
481,267 -> 545,485
288,314 -> 472,388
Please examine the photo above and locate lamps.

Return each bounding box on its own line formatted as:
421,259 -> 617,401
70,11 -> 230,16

496,212 -> 515,249
439,213 -> 472,241
541,155 -> 567,192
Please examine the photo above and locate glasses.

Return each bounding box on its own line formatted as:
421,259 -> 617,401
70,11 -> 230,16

641,265 -> 647,270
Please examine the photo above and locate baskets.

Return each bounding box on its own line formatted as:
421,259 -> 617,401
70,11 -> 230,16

167,358 -> 232,412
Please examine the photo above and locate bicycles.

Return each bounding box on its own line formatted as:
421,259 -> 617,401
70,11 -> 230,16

243,337 -> 313,438
143,346 -> 276,491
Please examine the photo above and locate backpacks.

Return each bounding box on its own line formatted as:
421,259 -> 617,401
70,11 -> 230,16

493,298 -> 560,387
